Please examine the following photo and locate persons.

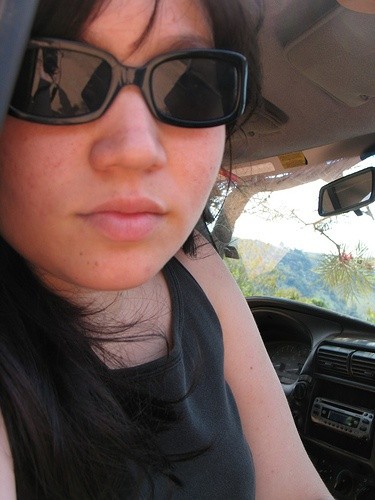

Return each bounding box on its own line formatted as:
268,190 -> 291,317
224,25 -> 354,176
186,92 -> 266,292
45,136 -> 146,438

1,1 -> 338,500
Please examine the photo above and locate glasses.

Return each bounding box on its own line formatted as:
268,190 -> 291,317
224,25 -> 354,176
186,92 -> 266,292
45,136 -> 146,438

7,38 -> 247,129
